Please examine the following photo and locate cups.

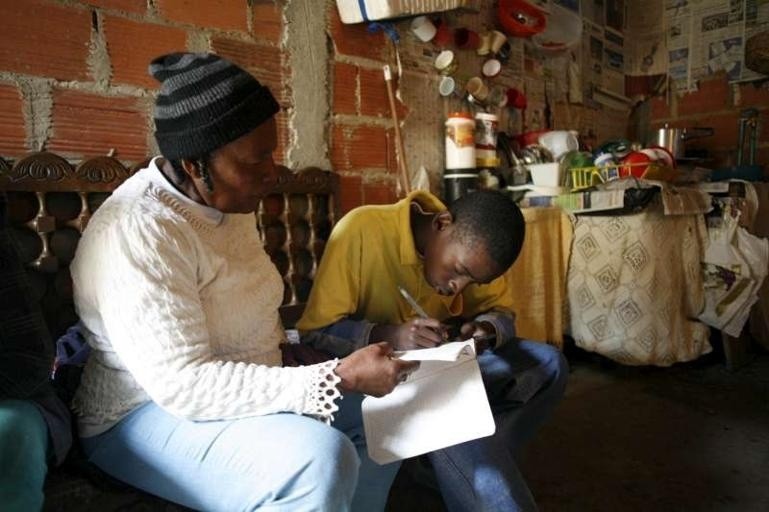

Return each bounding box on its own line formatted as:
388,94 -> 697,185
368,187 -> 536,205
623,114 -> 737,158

455,28 -> 481,51
482,59 -> 502,77
466,77 -> 488,100
430,19 -> 452,46
506,88 -> 526,108
538,129 -> 579,157
434,50 -> 459,76
477,31 -> 512,66
490,85 -> 508,108
440,78 -> 466,99
411,16 -> 436,43
594,152 -> 620,182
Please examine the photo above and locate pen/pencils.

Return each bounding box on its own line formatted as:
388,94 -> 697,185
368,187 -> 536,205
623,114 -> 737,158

397,285 -> 449,343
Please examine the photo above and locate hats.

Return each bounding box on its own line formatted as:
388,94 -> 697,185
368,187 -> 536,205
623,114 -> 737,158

148,51 -> 282,162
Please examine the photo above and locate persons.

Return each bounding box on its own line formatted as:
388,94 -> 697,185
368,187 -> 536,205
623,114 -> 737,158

293,187 -> 571,511
70,50 -> 422,511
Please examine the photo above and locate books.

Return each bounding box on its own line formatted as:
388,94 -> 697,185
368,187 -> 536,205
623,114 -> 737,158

360,336 -> 496,466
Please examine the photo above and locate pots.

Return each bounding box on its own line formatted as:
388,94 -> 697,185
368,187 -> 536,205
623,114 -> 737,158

658,125 -> 687,159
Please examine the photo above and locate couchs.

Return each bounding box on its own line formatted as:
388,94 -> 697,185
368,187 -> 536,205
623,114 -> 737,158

0,154 -> 342,512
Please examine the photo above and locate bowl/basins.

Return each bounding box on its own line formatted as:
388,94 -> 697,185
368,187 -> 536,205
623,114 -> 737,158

619,147 -> 676,182
531,11 -> 583,51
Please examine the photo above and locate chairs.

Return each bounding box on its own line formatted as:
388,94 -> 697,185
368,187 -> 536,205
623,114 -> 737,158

698,167 -> 768,372
